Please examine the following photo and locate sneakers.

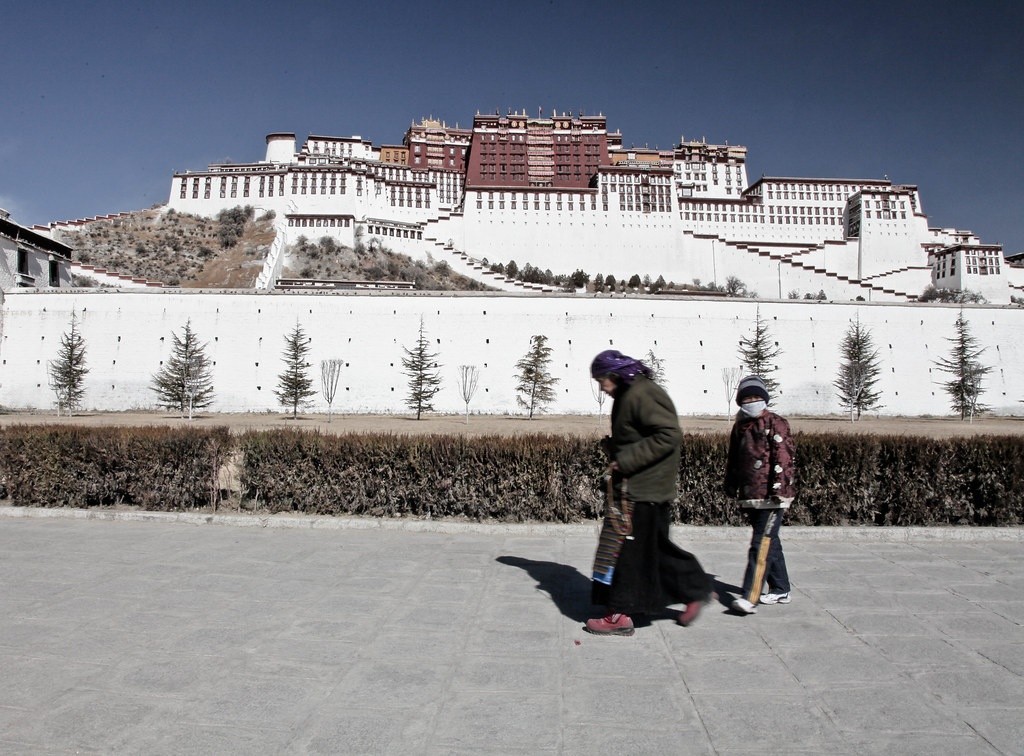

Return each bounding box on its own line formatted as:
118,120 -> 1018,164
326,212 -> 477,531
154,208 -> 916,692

759,592 -> 791,604
733,599 -> 758,614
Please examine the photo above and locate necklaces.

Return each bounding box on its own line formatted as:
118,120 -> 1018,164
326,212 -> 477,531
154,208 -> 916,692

607,462 -> 631,535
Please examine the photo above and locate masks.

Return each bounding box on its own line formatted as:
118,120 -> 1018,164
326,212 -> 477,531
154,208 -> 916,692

742,400 -> 767,418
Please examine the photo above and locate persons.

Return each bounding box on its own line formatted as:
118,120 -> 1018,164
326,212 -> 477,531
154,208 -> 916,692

582,350 -> 718,637
723,373 -> 797,614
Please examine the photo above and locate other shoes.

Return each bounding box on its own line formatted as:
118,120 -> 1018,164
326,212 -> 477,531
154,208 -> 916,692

587,613 -> 635,637
679,599 -> 706,626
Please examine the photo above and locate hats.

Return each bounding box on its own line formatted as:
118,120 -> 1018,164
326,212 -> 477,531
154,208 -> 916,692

736,375 -> 770,407
